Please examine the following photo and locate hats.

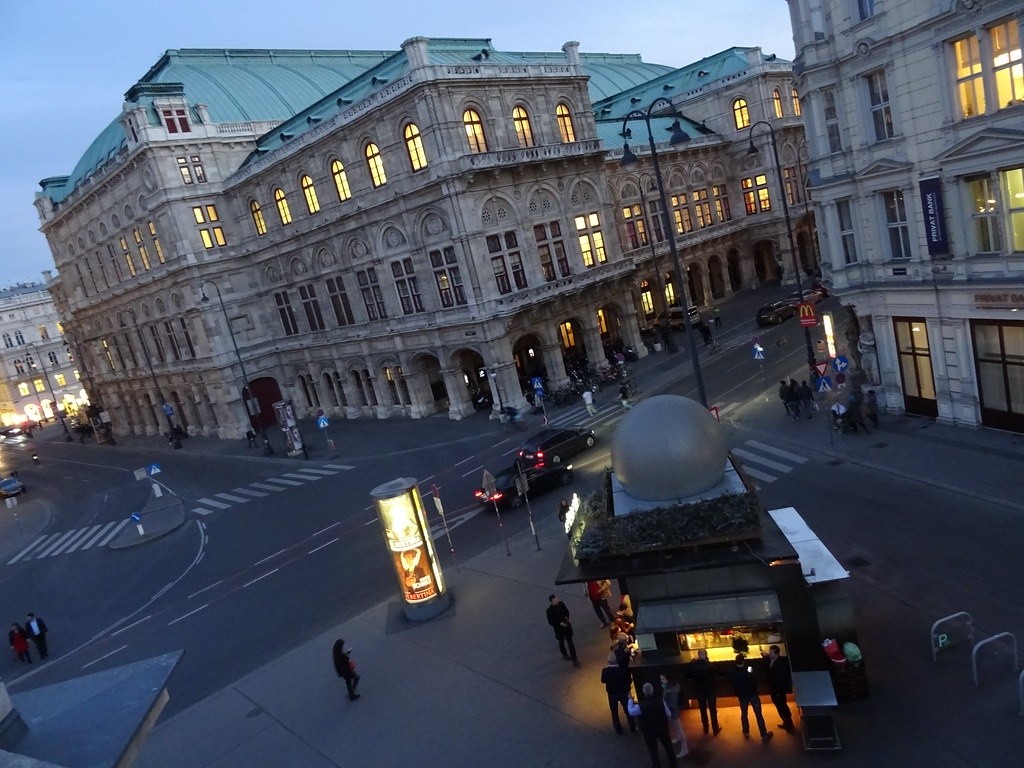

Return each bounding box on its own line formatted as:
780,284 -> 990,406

844,642 -> 861,661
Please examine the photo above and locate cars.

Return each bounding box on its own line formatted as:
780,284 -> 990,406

473,465 -> 573,511
515,428 -> 596,467
783,282 -> 831,312
0,477 -> 25,499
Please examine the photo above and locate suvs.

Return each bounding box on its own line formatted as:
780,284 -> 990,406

756,299 -> 798,326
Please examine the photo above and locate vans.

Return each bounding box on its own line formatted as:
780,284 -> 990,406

653,306 -> 701,332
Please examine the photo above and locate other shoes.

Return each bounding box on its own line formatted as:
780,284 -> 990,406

565,653 -> 571,659
715,724 -> 722,737
807,416 -> 813,419
778,721 -> 786,728
791,415 -> 799,422
763,731 -> 773,745
28,660 -> 32,664
600,622 -> 605,629
574,659 -> 582,666
351,694 -> 360,701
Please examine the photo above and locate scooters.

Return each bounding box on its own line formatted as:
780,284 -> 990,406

546,362 -> 631,410
618,96 -> 707,410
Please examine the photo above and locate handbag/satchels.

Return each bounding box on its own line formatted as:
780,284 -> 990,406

811,401 -> 819,411
348,659 -> 356,669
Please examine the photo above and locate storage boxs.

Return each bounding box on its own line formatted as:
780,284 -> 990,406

840,630 -> 870,698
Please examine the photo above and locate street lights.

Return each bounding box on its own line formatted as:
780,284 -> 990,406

746,120 -> 820,385
119,309 -> 181,443
24,344 -> 74,442
200,279 -> 275,458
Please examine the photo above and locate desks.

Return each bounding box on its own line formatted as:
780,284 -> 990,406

791,670 -> 843,753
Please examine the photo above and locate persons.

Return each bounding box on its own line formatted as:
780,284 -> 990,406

713,306 -> 721,327
333,638 -> 361,701
167,424 -> 189,448
246,429 -> 259,449
627,668 -> 688,768
780,379 -> 877,434
699,322 -> 712,345
546,594 -> 580,665
583,391 -> 597,416
761,645 -> 794,732
727,654 -> 773,743
22,421 -> 91,461
8,613 -> 48,663
559,499 -> 573,541
582,579 -> 638,736
685,648 -> 722,736
662,328 -> 674,352
617,383 -> 632,413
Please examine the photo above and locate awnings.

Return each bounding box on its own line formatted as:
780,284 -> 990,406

768,506 -> 851,583
